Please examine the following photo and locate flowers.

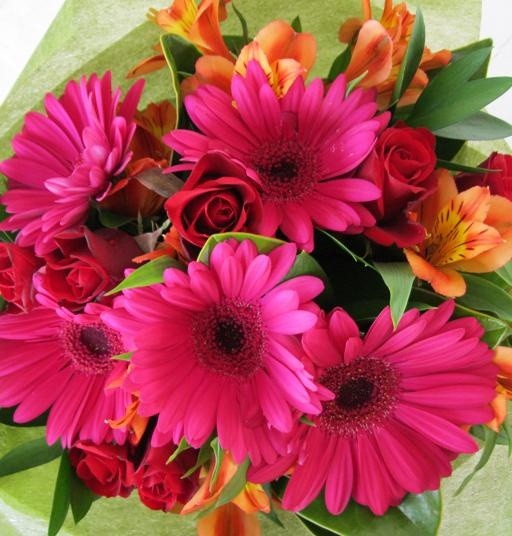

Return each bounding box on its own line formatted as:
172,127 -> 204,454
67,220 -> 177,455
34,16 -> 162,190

2,1 -> 512,536
